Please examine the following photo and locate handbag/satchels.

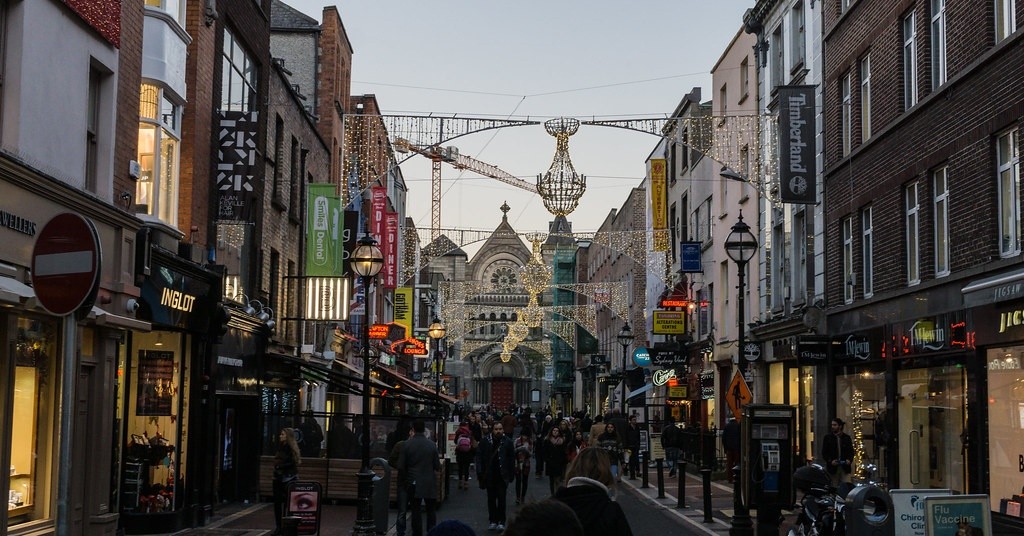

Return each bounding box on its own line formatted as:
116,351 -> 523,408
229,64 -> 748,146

475,457 -> 492,488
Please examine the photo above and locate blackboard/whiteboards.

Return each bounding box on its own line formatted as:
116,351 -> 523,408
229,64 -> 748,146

638,430 -> 648,457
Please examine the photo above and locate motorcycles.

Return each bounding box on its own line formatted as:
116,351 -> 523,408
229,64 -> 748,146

784,464 -> 857,536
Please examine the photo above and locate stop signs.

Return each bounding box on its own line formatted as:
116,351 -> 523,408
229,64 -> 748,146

31,210 -> 99,317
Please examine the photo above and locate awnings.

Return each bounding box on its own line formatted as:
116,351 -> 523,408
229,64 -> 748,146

376,361 -> 459,404
334,359 -> 395,389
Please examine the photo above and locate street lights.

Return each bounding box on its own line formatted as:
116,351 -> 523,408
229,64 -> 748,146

349,230 -> 386,536
724,207 -> 759,536
428,314 -> 446,418
616,321 -> 636,417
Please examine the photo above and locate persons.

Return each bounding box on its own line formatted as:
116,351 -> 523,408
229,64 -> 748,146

505,499 -> 582,536
548,447 -> 634,536
390,428 -> 412,536
955,519 -> 984,536
454,400 -> 640,505
651,415 -> 662,432
661,416 -> 683,479
402,416 -> 441,536
823,417 -> 854,482
270,427 -> 301,531
429,520 -> 477,536
721,420 -> 741,483
477,422 -> 516,531
299,410 -> 325,455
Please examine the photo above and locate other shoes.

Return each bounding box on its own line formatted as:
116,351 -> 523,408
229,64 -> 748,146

497,524 -> 505,532
488,523 -> 497,531
270,528 -> 282,536
536,474 -> 540,479
636,471 -> 643,478
669,471 -> 677,480
516,497 -> 521,504
541,474 -> 545,479
520,497 -> 526,504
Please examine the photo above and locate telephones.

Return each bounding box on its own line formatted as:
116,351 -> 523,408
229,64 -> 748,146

761,450 -> 780,472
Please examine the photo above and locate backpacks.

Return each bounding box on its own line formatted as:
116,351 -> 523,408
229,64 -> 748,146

458,433 -> 471,454
